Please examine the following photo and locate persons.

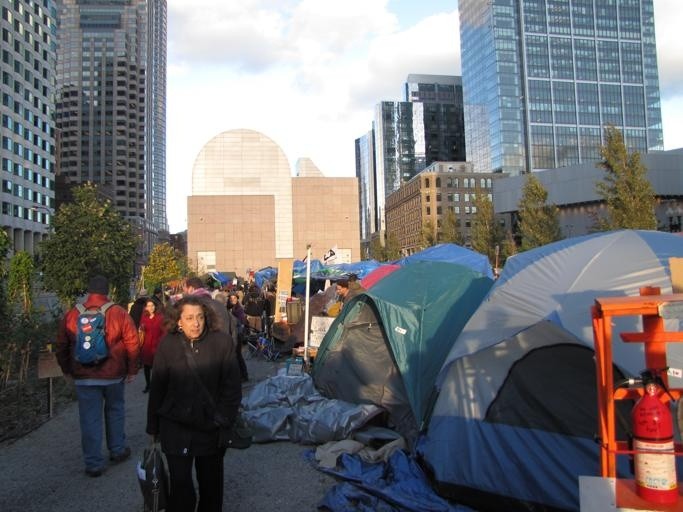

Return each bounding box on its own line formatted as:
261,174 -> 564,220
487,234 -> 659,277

146,296 -> 242,511
138,278 -> 277,393
55,276 -> 140,478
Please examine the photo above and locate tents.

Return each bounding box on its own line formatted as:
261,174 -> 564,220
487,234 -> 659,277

252,229 -> 683,511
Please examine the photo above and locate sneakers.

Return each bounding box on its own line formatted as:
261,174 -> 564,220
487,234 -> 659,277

85,465 -> 103,477
110,447 -> 130,461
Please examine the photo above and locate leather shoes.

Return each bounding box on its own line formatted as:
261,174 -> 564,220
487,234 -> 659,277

241,372 -> 248,382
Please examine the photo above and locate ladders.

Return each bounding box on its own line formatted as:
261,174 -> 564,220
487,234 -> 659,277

591,294 -> 683,477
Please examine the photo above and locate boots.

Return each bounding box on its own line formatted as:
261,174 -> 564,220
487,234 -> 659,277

142,384 -> 149,393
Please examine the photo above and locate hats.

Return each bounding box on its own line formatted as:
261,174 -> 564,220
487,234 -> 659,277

154,288 -> 161,293
88,275 -> 109,294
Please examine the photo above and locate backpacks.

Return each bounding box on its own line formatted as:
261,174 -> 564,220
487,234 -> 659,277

75,310 -> 107,367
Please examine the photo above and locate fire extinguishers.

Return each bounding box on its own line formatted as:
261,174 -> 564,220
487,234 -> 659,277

616,367 -> 679,505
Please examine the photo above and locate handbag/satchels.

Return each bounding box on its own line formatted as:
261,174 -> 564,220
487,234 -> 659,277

217,427 -> 252,449
138,327 -> 145,346
137,448 -> 169,511
